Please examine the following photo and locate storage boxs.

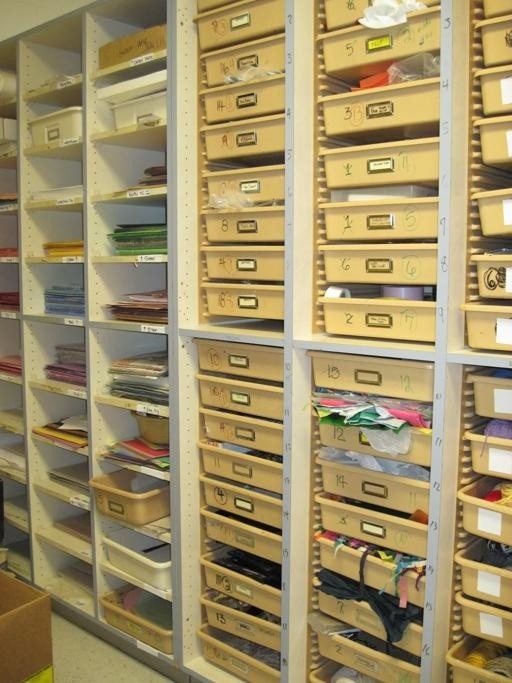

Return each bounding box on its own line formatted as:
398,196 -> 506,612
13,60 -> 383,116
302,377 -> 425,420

0,571 -> 54,683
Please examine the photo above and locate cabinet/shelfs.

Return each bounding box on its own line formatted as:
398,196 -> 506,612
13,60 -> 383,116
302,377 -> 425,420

0,2 -> 169,675
170,1 -> 512,681
